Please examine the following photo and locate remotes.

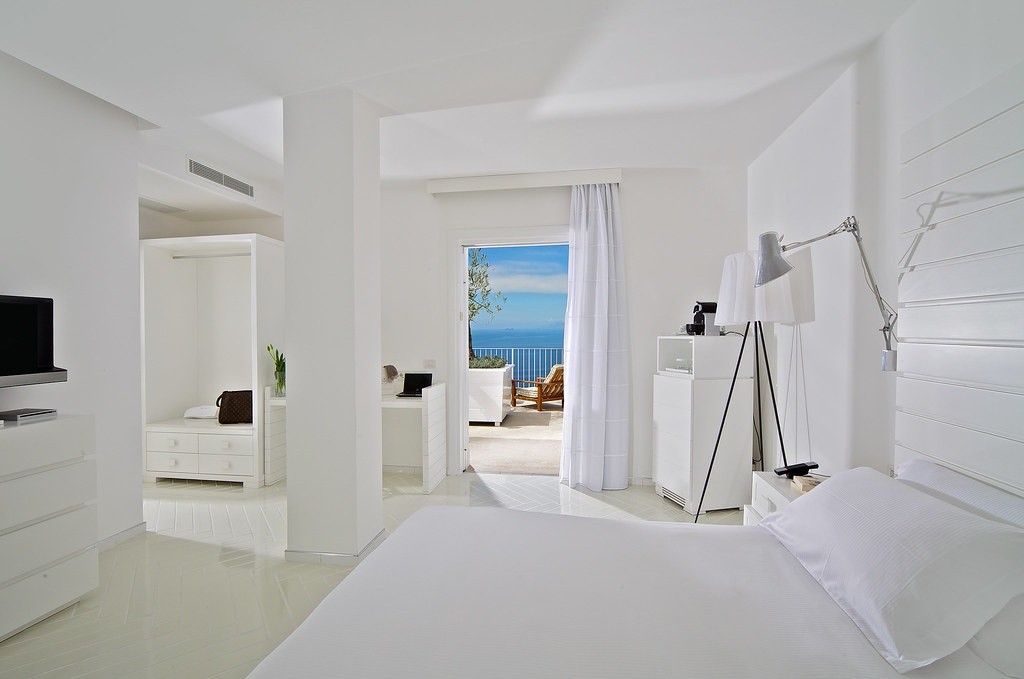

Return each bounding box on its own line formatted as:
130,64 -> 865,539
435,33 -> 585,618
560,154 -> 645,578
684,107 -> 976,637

774,461 -> 819,476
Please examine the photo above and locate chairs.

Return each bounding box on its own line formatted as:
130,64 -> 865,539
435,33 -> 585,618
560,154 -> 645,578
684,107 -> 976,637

510,362 -> 566,413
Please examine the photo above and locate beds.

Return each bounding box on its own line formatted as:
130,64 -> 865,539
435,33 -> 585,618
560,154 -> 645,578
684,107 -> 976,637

242,437 -> 1024,679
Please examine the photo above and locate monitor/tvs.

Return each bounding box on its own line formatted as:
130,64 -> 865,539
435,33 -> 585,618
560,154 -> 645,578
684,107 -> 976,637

0,295 -> 54,377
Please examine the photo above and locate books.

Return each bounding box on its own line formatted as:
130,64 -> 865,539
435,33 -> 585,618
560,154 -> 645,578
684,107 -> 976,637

790,475 -> 820,495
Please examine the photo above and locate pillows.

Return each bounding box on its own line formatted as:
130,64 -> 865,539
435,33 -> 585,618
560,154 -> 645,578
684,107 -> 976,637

760,462 -> 1024,674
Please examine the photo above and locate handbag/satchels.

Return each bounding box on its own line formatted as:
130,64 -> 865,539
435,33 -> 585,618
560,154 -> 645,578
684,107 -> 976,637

217,390 -> 254,424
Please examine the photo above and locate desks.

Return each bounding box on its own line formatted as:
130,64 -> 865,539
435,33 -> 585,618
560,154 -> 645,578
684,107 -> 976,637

263,380 -> 451,489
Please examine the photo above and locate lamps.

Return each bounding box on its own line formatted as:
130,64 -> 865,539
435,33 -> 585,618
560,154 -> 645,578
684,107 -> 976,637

691,250 -> 791,524
748,216 -> 900,372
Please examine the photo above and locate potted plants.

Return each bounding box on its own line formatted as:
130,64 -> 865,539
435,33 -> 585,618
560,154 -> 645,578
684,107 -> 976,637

266,343 -> 286,398
468,249 -> 514,428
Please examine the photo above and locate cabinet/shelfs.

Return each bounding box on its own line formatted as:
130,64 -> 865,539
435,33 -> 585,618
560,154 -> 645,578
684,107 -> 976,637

0,407 -> 105,644
145,422 -> 253,491
646,332 -> 757,512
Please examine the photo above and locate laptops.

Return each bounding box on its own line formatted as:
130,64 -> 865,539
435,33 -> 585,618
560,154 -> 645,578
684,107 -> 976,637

395,374 -> 433,397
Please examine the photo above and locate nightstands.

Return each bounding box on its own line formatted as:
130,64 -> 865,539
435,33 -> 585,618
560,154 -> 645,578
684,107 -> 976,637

734,468 -> 830,527
